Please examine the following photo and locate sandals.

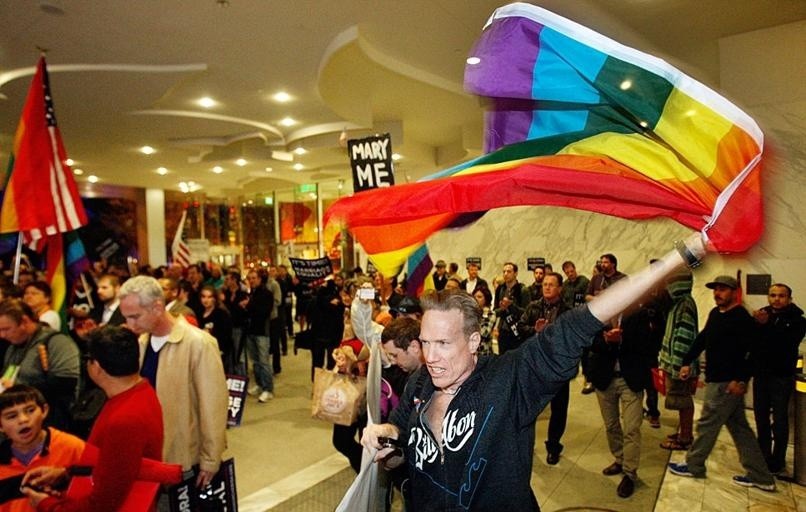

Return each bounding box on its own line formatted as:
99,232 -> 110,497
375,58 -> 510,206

660,433 -> 694,450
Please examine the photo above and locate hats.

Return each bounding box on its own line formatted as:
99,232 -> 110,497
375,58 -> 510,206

705,276 -> 737,288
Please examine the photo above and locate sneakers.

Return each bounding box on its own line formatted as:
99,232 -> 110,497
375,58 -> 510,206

617,475 -> 634,498
258,390 -> 275,403
732,475 -> 776,491
581,382 -> 594,394
247,385 -> 263,396
603,462 -> 622,475
647,415 -> 660,427
668,462 -> 694,477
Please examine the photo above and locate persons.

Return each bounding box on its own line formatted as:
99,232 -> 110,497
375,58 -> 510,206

3,251 -> 365,512
330,251 -> 806,512
359,229 -> 709,512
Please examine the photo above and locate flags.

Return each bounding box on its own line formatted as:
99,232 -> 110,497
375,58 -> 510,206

311,3 -> 770,261
171,207 -> 191,268
1,54 -> 131,336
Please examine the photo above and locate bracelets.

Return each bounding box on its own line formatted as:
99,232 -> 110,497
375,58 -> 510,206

675,239 -> 704,271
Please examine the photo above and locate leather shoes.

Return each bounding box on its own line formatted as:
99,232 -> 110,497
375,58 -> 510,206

547,452 -> 559,464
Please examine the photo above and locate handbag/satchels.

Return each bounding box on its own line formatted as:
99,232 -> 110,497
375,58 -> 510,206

311,357 -> 367,426
